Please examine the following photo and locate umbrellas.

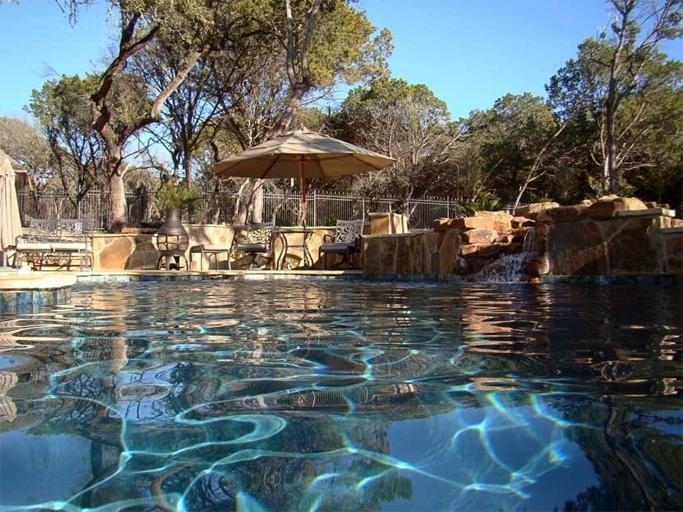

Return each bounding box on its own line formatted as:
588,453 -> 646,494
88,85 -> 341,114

0,145 -> 24,270
209,120 -> 397,266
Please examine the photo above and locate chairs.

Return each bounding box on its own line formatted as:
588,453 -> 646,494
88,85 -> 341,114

319,219 -> 364,270
233,223 -> 274,270
189,228 -> 236,271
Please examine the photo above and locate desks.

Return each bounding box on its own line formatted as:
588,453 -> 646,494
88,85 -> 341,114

367,212 -> 408,235
278,228 -> 317,270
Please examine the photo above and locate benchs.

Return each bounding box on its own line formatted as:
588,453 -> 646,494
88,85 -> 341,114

14,235 -> 94,271
